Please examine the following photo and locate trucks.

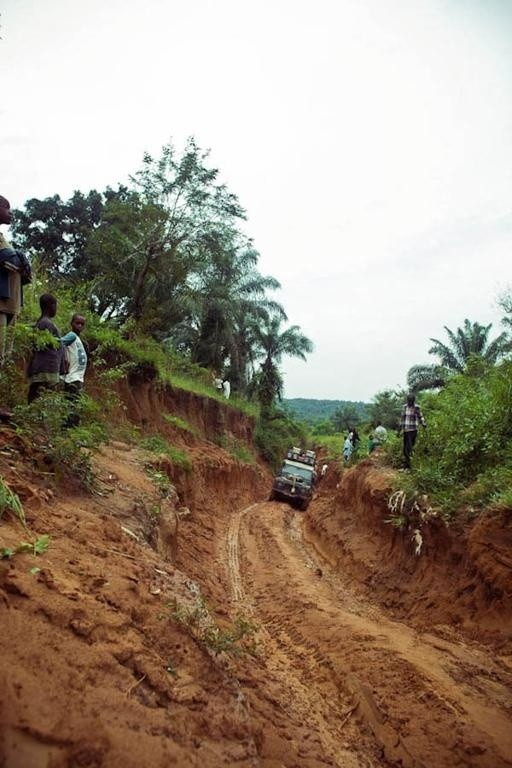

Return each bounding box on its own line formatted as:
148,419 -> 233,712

268,446 -> 317,510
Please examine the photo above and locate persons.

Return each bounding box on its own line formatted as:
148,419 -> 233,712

396,393 -> 428,467
223,378 -> 232,400
340,418 -> 390,465
214,375 -> 224,391
29,293 -> 70,407
61,315 -> 88,428
